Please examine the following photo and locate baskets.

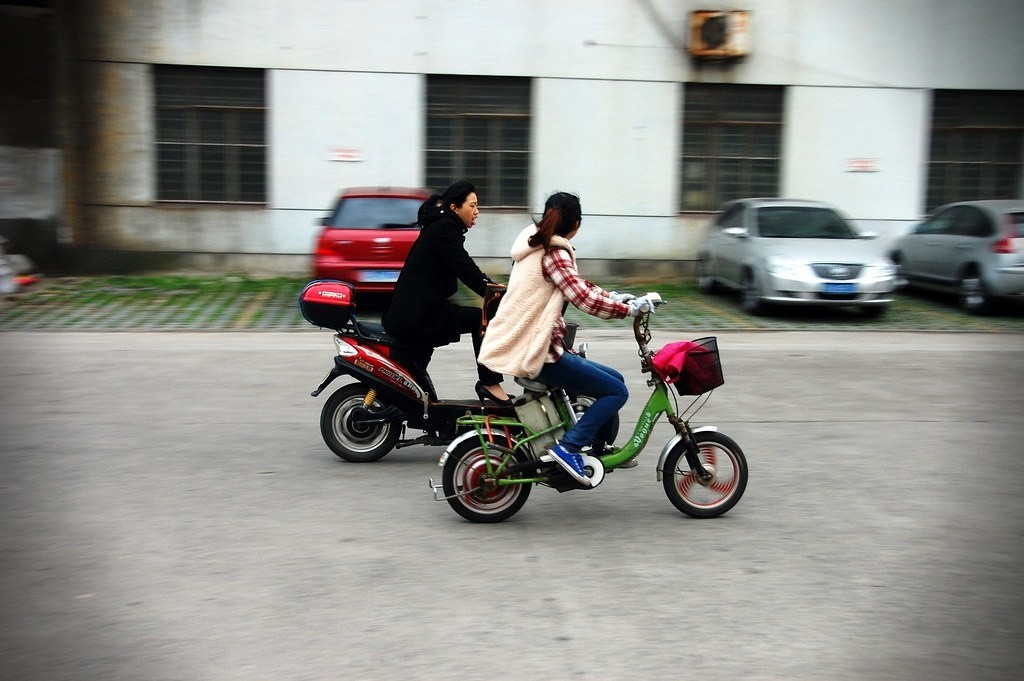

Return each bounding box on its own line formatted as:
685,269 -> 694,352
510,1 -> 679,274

669,337 -> 725,397
563,319 -> 580,352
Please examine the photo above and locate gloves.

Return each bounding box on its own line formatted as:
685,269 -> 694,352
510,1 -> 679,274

610,290 -> 635,303
630,298 -> 655,316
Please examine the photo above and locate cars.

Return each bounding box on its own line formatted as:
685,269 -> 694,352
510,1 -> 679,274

695,200 -> 900,322
307,183 -> 437,303
887,200 -> 1024,309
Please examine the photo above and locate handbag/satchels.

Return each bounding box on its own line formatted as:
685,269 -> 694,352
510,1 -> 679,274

653,341 -> 717,386
482,281 -> 508,328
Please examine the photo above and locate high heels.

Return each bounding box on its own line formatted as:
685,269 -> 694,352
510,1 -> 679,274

474,381 -> 516,408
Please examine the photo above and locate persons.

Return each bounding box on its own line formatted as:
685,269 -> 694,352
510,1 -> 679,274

476,190 -> 647,487
381,180 -> 518,406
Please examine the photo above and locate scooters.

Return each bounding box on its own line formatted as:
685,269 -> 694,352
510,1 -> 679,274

298,278 -> 601,464
428,296 -> 749,524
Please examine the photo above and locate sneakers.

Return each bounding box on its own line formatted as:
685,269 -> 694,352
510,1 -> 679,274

545,443 -> 594,487
598,448 -> 637,469
